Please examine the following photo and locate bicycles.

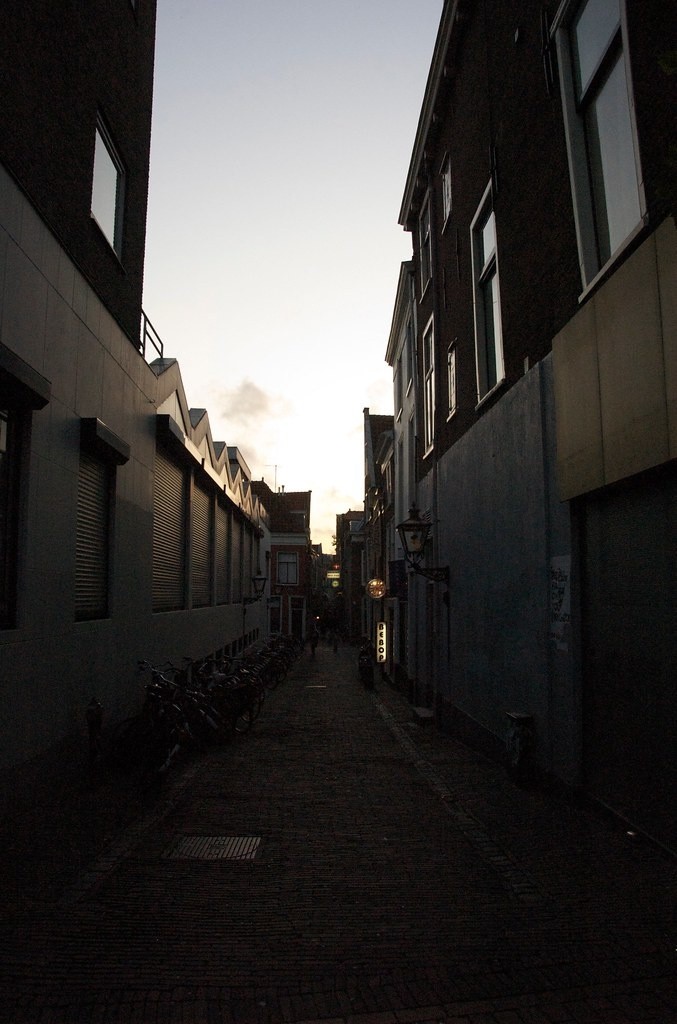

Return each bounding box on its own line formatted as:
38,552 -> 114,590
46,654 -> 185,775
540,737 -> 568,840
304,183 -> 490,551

116,625 -> 305,771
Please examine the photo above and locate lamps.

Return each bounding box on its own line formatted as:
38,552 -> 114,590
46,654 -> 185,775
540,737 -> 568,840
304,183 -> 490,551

243,567 -> 268,614
394,502 -> 448,584
